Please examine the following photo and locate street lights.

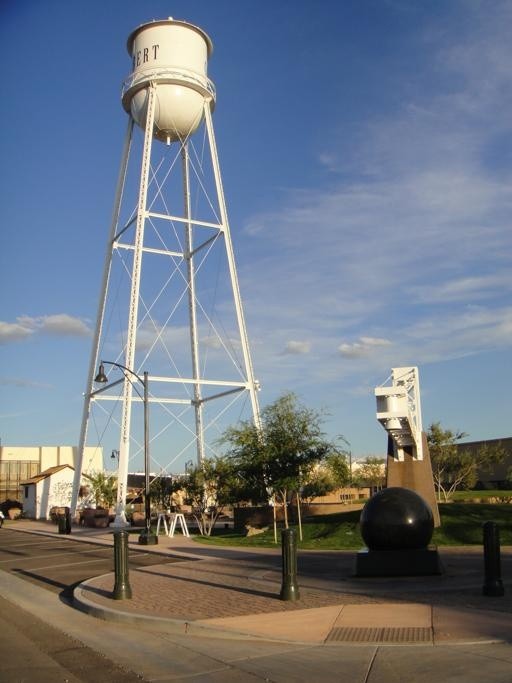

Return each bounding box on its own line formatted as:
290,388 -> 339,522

95,360 -> 158,544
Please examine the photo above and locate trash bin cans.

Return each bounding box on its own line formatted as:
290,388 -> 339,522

58,519 -> 70,534
